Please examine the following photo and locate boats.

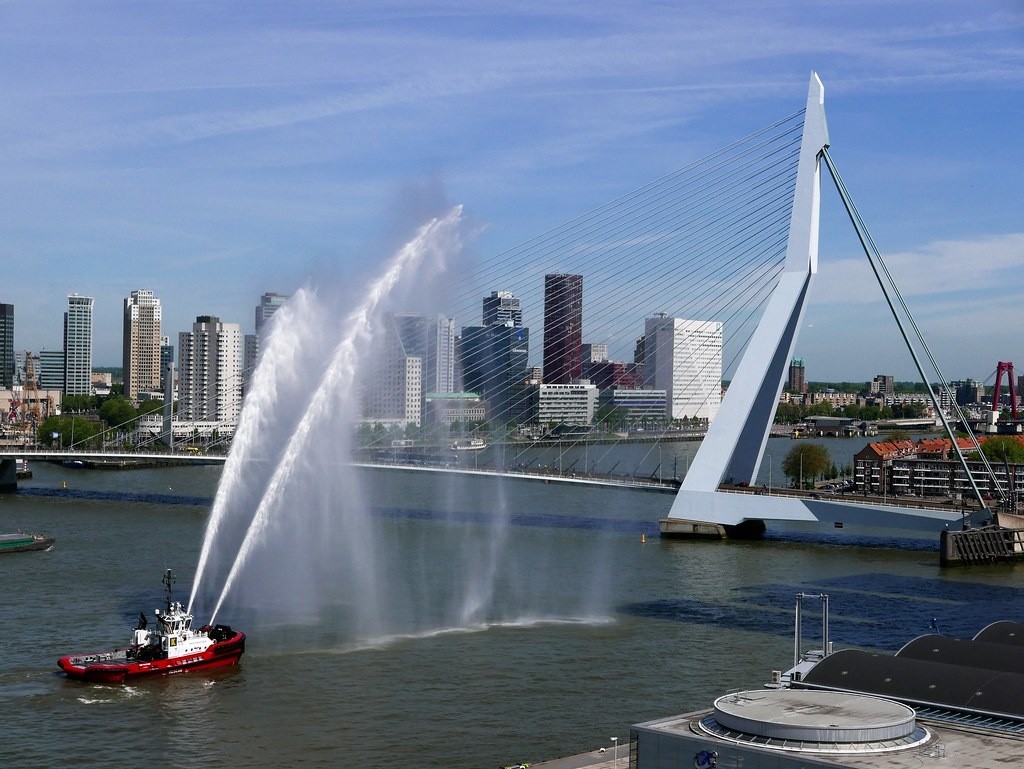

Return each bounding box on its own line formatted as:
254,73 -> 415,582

449,437 -> 487,450
55,567 -> 249,685
0,531 -> 59,553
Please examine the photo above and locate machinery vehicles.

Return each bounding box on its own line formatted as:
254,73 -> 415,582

2,350 -> 57,437
